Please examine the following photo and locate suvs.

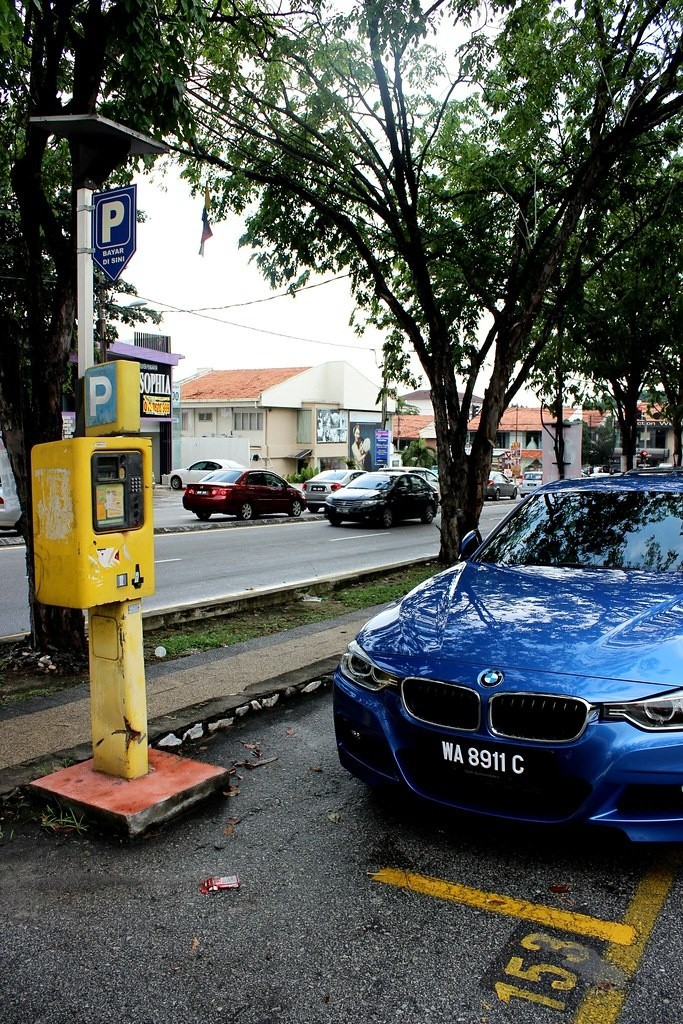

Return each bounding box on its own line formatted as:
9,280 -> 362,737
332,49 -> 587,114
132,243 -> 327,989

519,471 -> 543,498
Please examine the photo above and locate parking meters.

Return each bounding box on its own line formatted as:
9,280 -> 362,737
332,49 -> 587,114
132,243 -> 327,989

20,360 -> 232,844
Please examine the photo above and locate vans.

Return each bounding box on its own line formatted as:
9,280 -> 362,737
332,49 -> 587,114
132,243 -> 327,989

378,468 -> 442,506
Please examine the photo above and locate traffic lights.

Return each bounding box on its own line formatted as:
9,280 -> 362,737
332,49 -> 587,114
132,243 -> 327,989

471,404 -> 479,417
641,450 -> 647,464
637,406 -> 642,420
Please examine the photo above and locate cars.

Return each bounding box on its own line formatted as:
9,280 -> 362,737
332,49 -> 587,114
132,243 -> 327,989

485,471 -> 518,501
169,459 -> 250,491
324,472 -> 439,528
302,469 -> 368,513
182,469 -> 307,521
330,466 -> 683,847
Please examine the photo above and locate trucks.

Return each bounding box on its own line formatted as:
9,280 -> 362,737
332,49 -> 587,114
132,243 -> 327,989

0,431 -> 23,537
